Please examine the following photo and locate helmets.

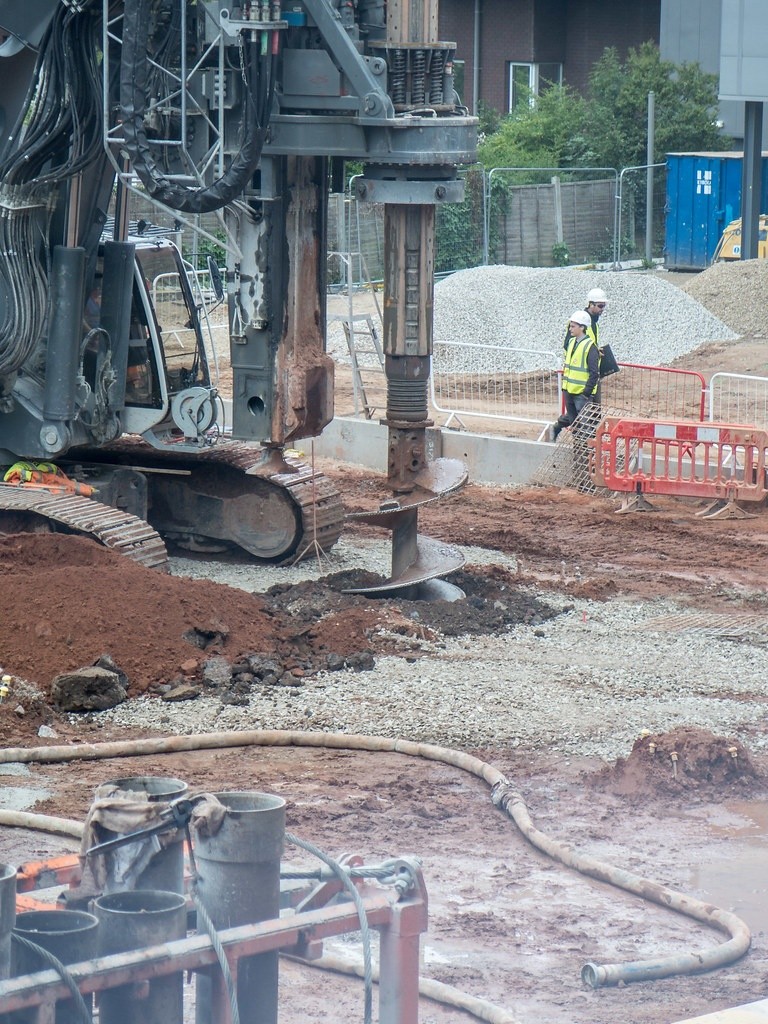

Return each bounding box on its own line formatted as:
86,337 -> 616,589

568,311 -> 592,328
586,288 -> 609,304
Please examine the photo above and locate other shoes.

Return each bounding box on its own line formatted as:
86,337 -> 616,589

548,422 -> 562,443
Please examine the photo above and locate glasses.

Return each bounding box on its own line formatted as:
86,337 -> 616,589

594,305 -> 605,308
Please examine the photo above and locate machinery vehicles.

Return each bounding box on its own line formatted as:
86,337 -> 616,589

2,0 -> 517,610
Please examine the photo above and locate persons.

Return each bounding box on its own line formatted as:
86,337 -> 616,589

538,288 -> 607,443
561,311 -> 599,449
